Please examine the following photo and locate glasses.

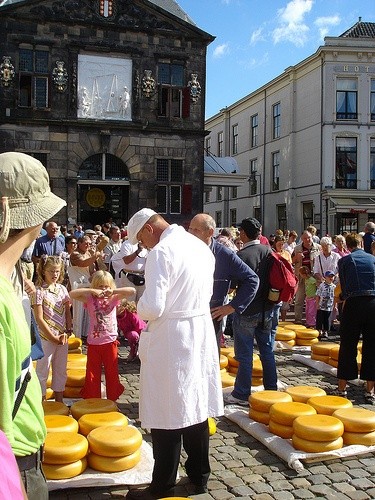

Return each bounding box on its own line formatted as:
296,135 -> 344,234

237,243 -> 243,244
69,242 -> 78,244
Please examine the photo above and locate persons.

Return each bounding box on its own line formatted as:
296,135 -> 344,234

213,226 -> 243,349
32,255 -> 73,404
325,232 -> 375,404
69,270 -> 138,403
0,151 -> 68,500
223,217 -> 279,405
189,214 -> 259,435
117,296 -> 149,361
123,208 -> 224,500
257,222 -> 375,337
9,220 -> 153,342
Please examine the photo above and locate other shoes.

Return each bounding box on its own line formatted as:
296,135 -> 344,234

364,390 -> 375,405
327,387 -> 348,399
175,477 -> 208,497
127,488 -> 170,500
318,332 -> 329,338
224,391 -> 249,406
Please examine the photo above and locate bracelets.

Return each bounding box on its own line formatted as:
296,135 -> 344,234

135,251 -> 141,258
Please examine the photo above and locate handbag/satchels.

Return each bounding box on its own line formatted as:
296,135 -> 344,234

119,270 -> 145,286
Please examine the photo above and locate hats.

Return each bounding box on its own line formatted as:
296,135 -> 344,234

85,229 -> 97,235
0,151 -> 67,244
127,208 -> 157,245
325,270 -> 335,276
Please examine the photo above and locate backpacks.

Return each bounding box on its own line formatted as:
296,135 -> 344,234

263,251 -> 299,304
